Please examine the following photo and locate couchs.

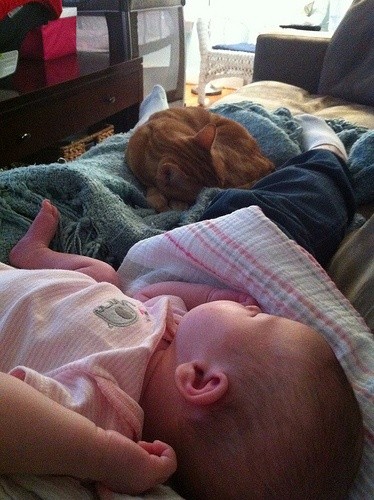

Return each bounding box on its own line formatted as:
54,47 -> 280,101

205,33 -> 366,127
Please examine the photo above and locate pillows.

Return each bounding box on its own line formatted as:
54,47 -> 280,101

317,0 -> 374,106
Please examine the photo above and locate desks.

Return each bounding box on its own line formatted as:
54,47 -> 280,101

0,53 -> 144,168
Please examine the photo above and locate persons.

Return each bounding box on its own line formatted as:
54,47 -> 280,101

0,199 -> 364,500
127,83 -> 356,268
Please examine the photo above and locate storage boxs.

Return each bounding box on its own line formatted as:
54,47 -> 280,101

20,7 -> 77,61
43,123 -> 114,163
63,0 -> 186,103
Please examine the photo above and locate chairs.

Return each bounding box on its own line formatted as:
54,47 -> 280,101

198,18 -> 255,106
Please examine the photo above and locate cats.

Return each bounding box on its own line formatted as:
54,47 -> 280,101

127,105 -> 276,212
